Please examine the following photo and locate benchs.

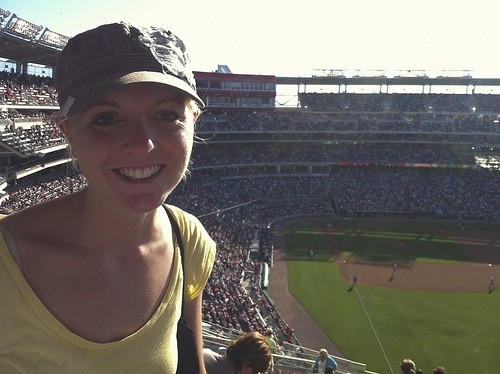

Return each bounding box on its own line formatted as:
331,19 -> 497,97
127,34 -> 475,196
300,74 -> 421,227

0,7 -> 500,374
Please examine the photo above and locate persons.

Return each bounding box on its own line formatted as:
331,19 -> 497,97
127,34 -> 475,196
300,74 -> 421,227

0,20 -> 217,373
324,365 -> 335,374
0,68 -> 90,217
202,331 -> 273,374
488,275 -> 496,295
353,273 -> 358,286
400,358 -> 416,374
433,366 -> 446,374
416,368 -> 423,374
162,90 -> 500,354
313,348 -> 338,374
393,264 -> 398,275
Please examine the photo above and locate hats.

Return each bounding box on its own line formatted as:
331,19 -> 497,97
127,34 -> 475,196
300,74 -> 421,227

55,22 -> 206,116
